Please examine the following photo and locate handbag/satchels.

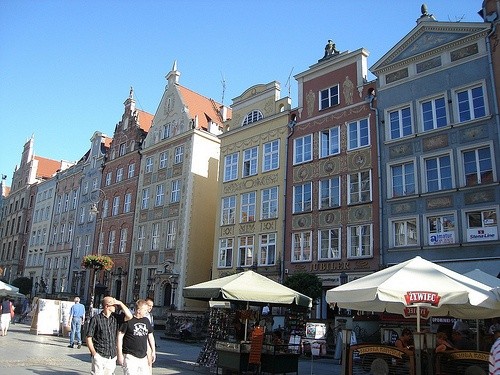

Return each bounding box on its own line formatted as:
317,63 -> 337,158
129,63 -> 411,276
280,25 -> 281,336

10,302 -> 14,318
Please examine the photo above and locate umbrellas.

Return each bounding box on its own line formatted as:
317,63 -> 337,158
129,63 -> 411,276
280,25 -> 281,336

441,269 -> 500,350
181,270 -> 314,347
324,253 -> 500,359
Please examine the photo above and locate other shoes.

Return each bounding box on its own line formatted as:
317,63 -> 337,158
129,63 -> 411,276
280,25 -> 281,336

68,346 -> 73,348
77,345 -> 81,349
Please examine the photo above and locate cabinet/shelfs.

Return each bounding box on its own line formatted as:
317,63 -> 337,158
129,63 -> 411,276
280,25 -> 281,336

216,340 -> 302,375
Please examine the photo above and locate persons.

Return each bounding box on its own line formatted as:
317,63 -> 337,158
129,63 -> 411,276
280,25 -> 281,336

0,295 -> 14,337
116,298 -> 157,375
86,297 -> 133,375
434,330 -> 458,353
31,281 -> 39,300
393,328 -> 416,375
68,295 -> 86,350
452,326 -> 500,375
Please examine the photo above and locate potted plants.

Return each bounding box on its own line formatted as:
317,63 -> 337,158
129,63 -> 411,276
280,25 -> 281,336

80,254 -> 115,272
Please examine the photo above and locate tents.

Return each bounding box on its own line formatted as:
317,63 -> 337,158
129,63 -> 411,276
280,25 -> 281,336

0,280 -> 26,300
